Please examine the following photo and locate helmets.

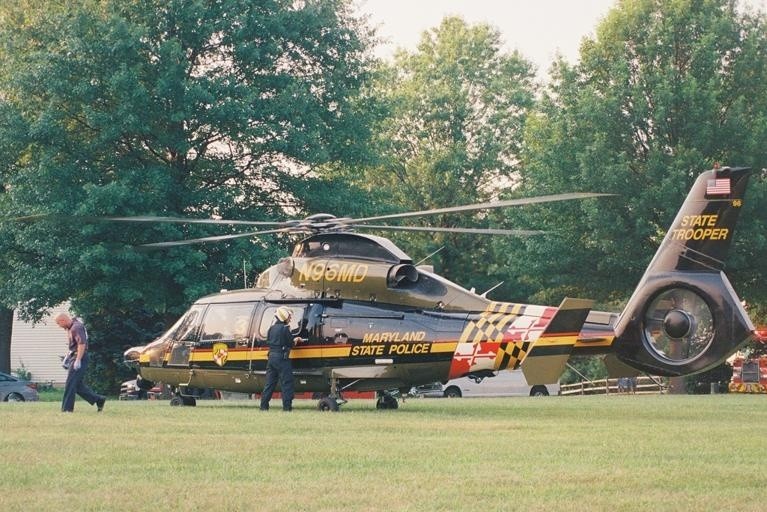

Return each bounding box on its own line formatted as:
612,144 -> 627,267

274,306 -> 293,322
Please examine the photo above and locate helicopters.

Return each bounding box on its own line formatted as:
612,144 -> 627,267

95,167 -> 756,409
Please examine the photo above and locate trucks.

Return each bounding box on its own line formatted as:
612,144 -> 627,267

148,380 -> 377,399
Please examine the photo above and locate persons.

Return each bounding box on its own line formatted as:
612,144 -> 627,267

54,313 -> 107,413
260,306 -> 305,411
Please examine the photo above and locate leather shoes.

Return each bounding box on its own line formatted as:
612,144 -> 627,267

97,396 -> 105,411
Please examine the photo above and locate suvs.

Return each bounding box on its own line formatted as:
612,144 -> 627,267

411,382 -> 446,398
118,374 -> 147,400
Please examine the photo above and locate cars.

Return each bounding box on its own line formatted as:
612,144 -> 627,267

0,372 -> 39,402
443,366 -> 562,396
